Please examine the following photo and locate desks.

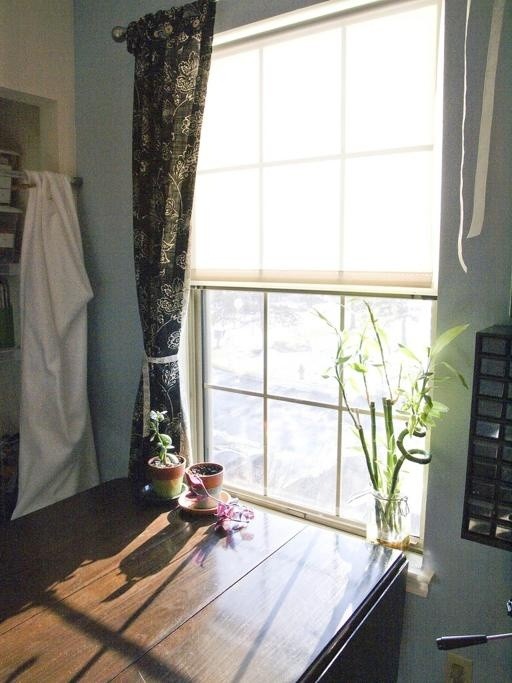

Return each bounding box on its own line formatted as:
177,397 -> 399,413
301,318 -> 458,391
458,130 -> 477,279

0,472 -> 414,681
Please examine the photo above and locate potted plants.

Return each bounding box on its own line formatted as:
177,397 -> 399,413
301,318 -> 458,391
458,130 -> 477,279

319,290 -> 468,553
137,405 -> 187,497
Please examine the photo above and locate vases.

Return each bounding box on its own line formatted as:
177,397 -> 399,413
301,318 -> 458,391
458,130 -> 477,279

186,462 -> 224,508
